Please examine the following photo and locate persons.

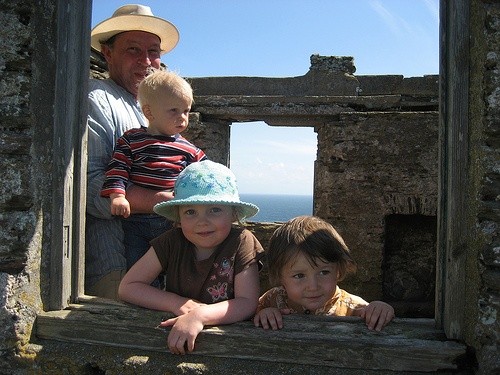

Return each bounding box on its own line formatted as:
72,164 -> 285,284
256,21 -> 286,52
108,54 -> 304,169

116,159 -> 268,357
253,214 -> 396,333
82,4 -> 181,304
97,70 -> 208,291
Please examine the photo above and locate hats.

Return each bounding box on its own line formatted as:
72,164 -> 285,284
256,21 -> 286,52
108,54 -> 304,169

153,159 -> 259,223
90,4 -> 180,55
